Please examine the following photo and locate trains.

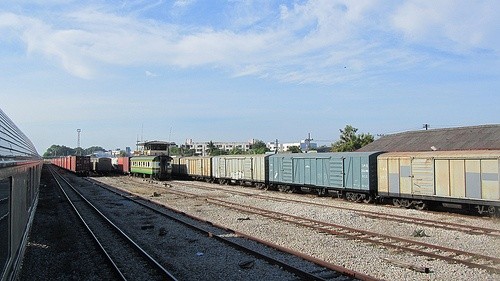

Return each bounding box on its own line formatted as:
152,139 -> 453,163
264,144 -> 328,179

49,156 -> 92,176
112,155 -> 176,181
171,149 -> 500,213
89,157 -> 114,176
0,110 -> 46,280
